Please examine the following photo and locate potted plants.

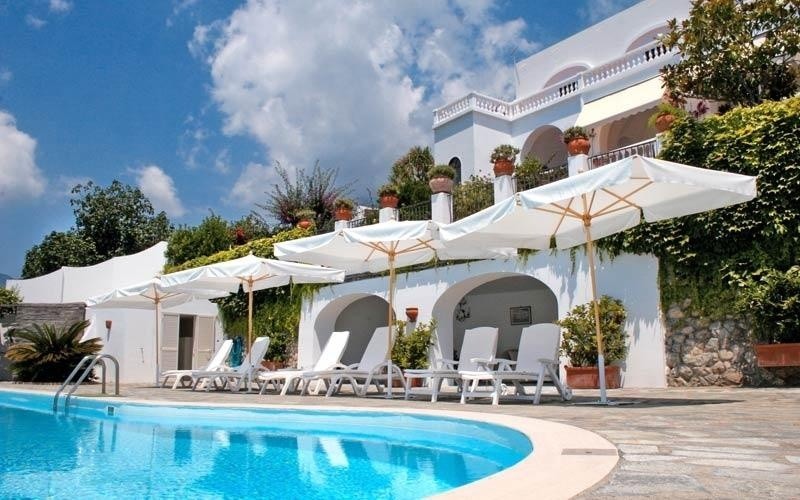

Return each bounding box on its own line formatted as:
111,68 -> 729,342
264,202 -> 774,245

727,262 -> 799,368
560,126 -> 592,156
387,315 -> 439,388
376,184 -> 400,208
559,293 -> 631,388
423,165 -> 456,192
489,142 -> 521,176
295,209 -> 317,229
333,199 -> 355,221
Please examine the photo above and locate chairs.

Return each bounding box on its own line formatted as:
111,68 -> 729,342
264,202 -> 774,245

259,329 -> 361,396
159,337 -> 238,391
458,319 -> 577,407
191,333 -> 280,390
398,323 -> 506,403
294,324 -> 409,397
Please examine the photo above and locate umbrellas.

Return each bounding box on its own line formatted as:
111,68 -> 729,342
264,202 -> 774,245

270,217 -> 521,400
84,276 -> 234,388
157,252 -> 346,395
438,152 -> 750,408
